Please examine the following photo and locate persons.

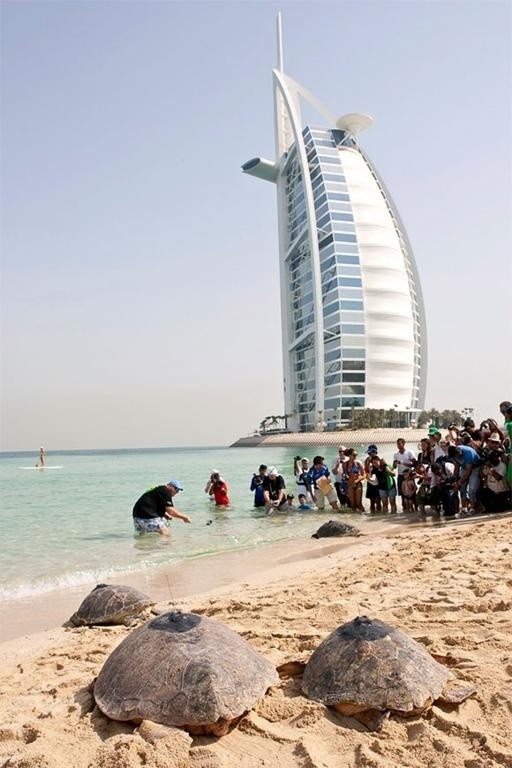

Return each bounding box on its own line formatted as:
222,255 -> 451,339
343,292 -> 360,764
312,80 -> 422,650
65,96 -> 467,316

251,401 -> 512,517
133,479 -> 192,538
204,469 -> 231,508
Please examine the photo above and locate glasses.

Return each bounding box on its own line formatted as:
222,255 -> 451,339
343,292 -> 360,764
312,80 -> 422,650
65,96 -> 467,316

175,488 -> 179,492
353,453 -> 358,456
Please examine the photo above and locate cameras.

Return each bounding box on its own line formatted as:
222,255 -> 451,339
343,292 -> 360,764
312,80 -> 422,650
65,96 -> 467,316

270,490 -> 278,500
342,474 -> 349,481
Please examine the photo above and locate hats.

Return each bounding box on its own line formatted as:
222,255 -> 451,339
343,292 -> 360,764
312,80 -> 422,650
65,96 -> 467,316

488,432 -> 501,443
209,469 -> 219,477
365,447 -> 376,453
168,480 -> 184,491
427,427 -> 440,436
